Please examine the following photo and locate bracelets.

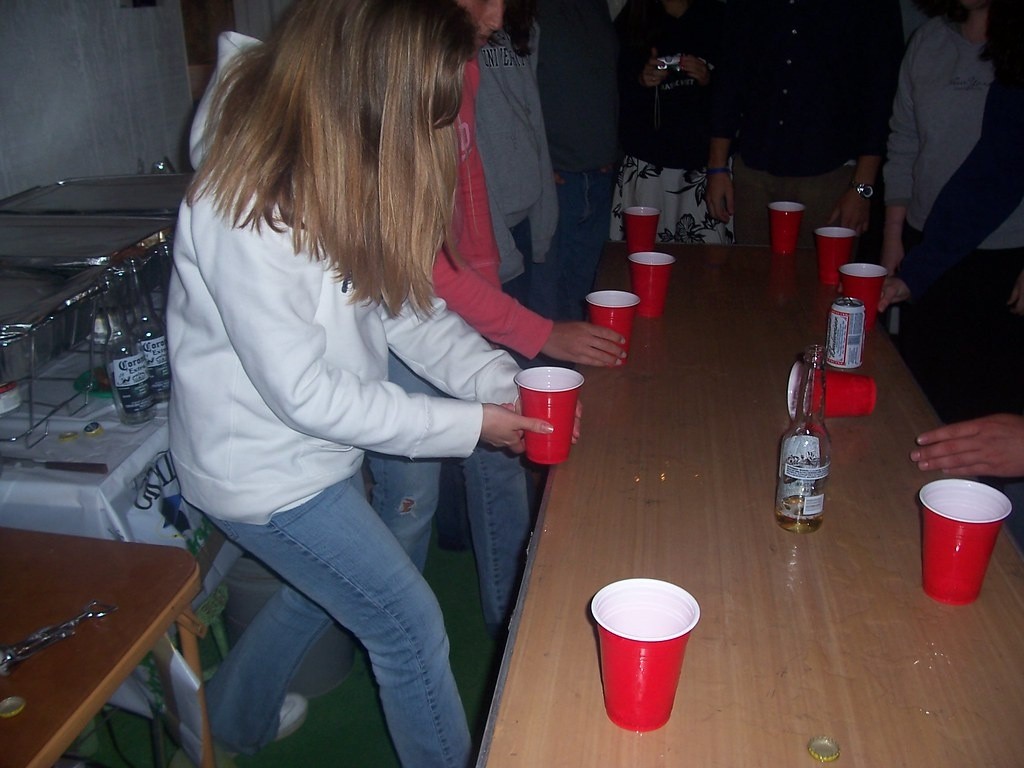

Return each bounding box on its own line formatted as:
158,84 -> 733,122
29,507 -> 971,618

706,167 -> 729,175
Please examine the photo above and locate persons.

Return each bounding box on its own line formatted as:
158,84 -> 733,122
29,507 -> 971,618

524,0 -> 619,372
609,0 -> 734,244
878,0 -> 1024,423
366,0 -> 627,636
166,0 -> 583,768
910,414 -> 1024,478
706,0 -> 898,247
434,0 -> 559,548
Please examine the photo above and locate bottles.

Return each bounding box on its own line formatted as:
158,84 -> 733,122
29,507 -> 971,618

776,343 -> 832,533
92,253 -> 171,424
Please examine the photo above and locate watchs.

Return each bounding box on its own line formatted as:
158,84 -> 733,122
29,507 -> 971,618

849,180 -> 874,199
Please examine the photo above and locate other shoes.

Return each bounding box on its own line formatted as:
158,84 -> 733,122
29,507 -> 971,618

271,690 -> 309,740
438,532 -> 473,550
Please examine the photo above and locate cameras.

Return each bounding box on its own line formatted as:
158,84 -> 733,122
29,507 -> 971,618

656,54 -> 682,72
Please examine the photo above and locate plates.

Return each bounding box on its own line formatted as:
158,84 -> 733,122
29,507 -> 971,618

74,365 -> 112,398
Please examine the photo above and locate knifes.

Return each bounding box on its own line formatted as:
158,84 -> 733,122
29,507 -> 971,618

0,457 -> 108,473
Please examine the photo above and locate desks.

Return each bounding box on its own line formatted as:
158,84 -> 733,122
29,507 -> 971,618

0,523 -> 216,768
474,234 -> 1024,768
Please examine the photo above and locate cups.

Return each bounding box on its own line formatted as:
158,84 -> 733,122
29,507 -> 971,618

816,227 -> 856,285
591,578 -> 701,733
514,367 -> 584,464
919,478 -> 1013,606
770,202 -> 804,254
788,363 -> 877,422
628,251 -> 675,317
624,206 -> 661,253
587,291 -> 641,367
840,263 -> 888,332
